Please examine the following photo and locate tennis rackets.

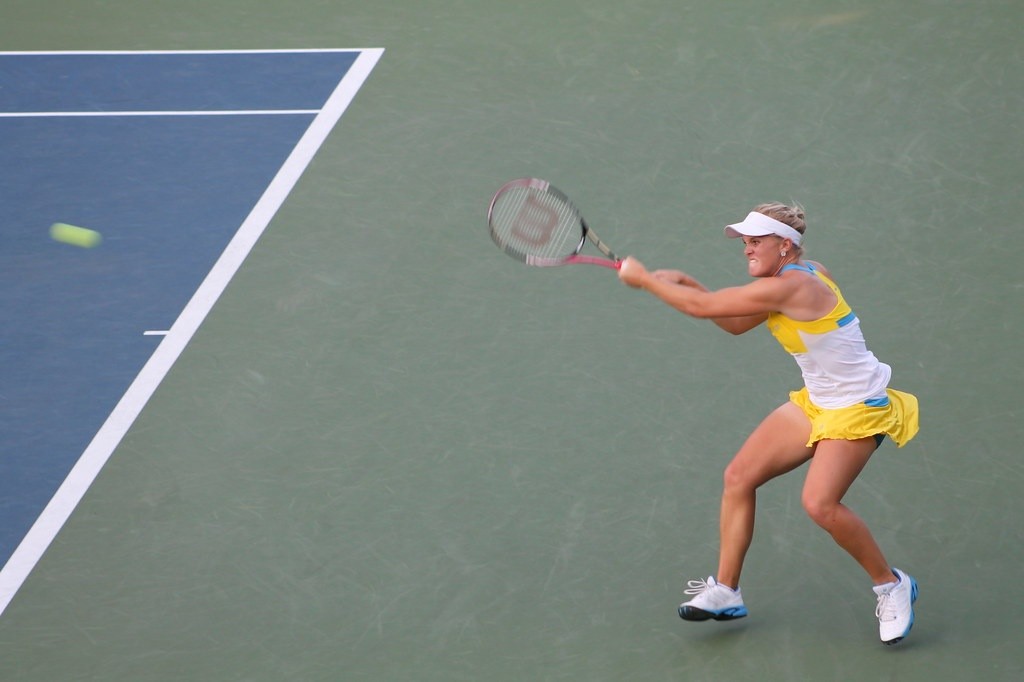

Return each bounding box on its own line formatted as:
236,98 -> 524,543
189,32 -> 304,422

486,176 -> 626,269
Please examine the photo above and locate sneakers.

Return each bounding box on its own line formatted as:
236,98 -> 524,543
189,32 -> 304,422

678,575 -> 748,621
871,567 -> 918,646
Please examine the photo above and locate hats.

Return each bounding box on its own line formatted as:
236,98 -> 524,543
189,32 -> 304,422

723,211 -> 803,249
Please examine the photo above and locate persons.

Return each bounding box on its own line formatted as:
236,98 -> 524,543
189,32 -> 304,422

614,202 -> 924,645
